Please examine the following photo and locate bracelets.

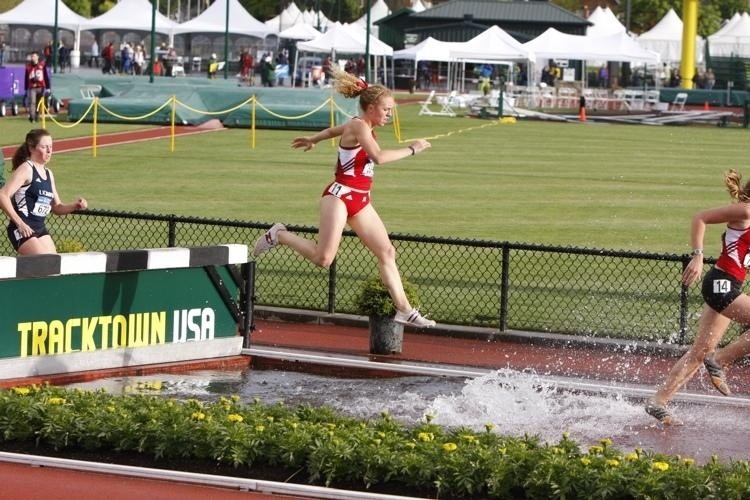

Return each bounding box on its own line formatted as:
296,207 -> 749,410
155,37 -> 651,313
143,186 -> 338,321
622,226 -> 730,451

408,146 -> 414,156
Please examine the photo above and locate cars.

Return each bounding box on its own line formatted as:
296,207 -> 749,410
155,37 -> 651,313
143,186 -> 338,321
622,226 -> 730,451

293,55 -> 323,83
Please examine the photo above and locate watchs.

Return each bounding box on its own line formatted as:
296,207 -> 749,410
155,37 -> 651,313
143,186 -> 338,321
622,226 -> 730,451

691,248 -> 704,255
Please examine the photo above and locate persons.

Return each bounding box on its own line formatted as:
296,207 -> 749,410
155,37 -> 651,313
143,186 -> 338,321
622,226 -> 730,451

238,47 -> 290,87
645,167 -> 750,426
0,129 -> 88,256
89,40 -> 176,76
24,52 -> 50,122
254,59 -> 436,328
208,53 -> 218,79
43,40 -> 72,73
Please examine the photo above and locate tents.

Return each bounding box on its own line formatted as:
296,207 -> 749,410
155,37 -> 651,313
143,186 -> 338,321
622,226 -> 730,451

391,24 -> 715,91
292,23 -> 392,88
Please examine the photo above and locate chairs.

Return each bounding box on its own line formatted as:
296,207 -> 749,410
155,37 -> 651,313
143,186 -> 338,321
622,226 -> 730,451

417,86 -> 688,117
192,57 -> 202,72
80,84 -> 102,99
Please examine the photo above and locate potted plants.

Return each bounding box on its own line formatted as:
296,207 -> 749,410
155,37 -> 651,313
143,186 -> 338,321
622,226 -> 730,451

353,272 -> 420,355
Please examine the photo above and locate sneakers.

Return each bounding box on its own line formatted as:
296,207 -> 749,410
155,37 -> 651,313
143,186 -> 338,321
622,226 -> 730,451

253,222 -> 286,257
644,400 -> 684,427
704,351 -> 731,395
393,308 -> 437,329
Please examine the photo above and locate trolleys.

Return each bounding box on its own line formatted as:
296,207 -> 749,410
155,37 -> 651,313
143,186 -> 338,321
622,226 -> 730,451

0,63 -> 64,118
